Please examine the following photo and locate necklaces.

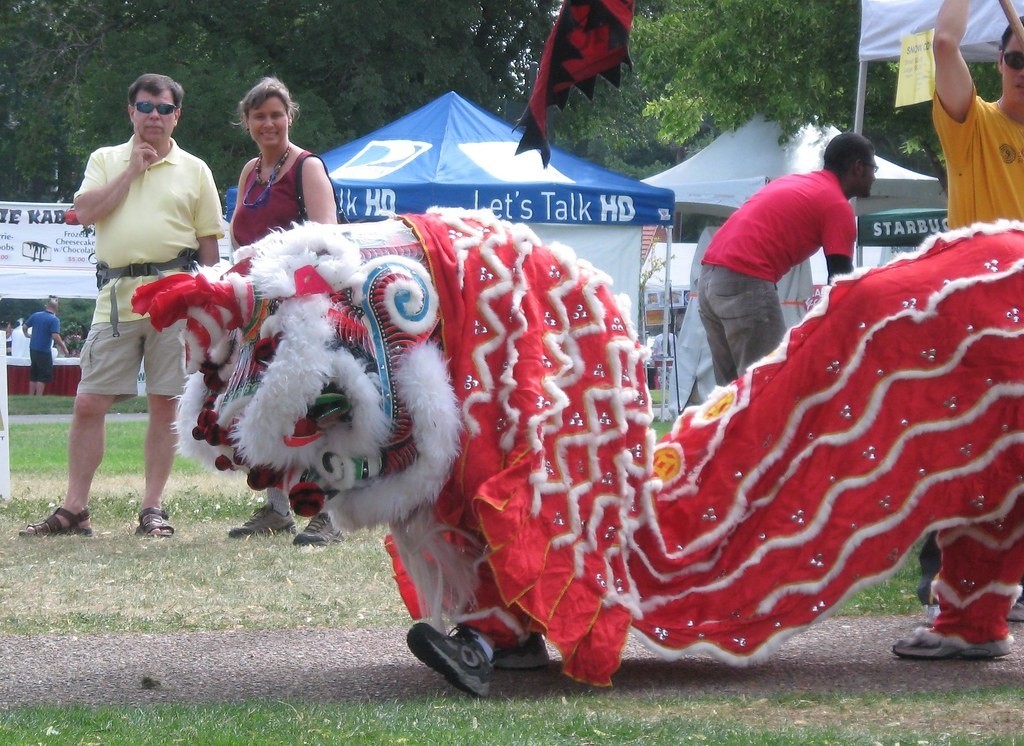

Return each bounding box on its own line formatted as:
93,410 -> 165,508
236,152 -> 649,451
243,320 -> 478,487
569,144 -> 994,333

243,144 -> 292,208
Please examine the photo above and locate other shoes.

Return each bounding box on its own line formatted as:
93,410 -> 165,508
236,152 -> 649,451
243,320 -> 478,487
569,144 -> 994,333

1006,602 -> 1024,621
927,605 -> 941,626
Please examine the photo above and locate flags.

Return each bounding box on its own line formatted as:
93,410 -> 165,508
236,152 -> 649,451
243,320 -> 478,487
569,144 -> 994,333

510,0 -> 635,171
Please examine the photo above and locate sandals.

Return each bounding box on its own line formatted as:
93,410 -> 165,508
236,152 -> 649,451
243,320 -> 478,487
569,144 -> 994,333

18,507 -> 92,537
135,507 -> 175,536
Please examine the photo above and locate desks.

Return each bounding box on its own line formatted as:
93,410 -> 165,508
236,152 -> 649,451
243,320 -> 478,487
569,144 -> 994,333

7,356 -> 81,397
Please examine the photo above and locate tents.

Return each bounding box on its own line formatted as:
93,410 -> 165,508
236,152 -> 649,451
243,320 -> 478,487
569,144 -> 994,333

221,90 -> 676,423
855,207 -> 949,268
636,104 -> 941,268
853,0 -> 1024,145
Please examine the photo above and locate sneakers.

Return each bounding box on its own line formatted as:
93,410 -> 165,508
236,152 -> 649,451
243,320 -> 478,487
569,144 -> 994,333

893,628 -> 1012,658
493,633 -> 549,669
229,502 -> 296,538
406,622 -> 497,699
293,513 -> 345,546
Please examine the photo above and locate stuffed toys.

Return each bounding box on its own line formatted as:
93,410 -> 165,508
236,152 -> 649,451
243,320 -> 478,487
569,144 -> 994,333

126,205 -> 1024,699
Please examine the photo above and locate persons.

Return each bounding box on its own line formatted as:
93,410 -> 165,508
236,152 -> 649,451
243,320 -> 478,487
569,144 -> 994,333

696,132 -> 879,387
22,295 -> 69,397
228,77 -> 344,547
916,0 -> 1024,626
0,317 -> 14,356
18,73 -> 226,540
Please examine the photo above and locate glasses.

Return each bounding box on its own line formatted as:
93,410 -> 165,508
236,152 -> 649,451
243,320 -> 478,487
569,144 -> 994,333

863,163 -> 879,173
243,175 -> 274,208
136,102 -> 175,114
1004,51 -> 1024,70
